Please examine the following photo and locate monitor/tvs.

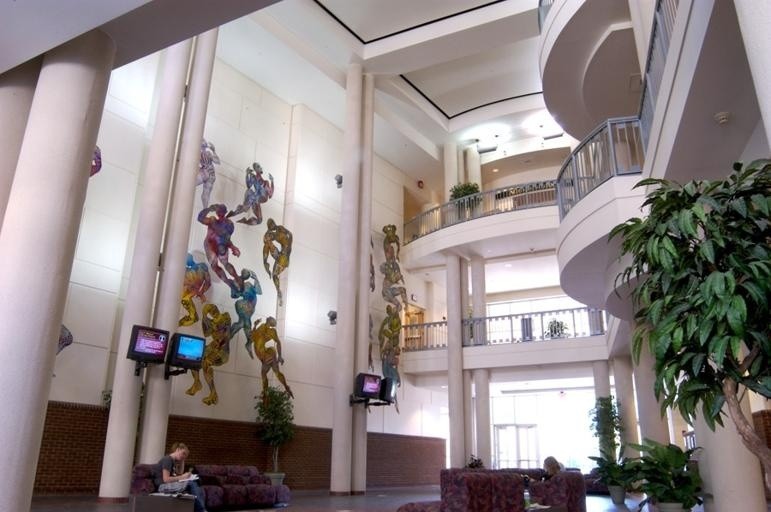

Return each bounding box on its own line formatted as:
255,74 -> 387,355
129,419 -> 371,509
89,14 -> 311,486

380,377 -> 398,403
354,372 -> 382,399
166,333 -> 206,370
126,324 -> 169,365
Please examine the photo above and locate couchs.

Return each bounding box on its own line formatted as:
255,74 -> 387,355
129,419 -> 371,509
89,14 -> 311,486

130,464 -> 292,511
395,466 -> 609,511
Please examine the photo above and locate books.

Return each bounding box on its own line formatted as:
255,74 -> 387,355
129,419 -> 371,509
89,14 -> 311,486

177,473 -> 200,483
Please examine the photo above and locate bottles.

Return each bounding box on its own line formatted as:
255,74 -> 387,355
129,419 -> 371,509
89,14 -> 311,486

523,488 -> 531,510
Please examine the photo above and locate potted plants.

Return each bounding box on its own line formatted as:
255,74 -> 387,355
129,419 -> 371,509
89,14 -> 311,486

584,436 -> 713,511
254,385 -> 299,486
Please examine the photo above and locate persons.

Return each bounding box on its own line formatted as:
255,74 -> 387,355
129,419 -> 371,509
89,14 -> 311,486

153,440 -> 209,511
522,456 -> 562,487
